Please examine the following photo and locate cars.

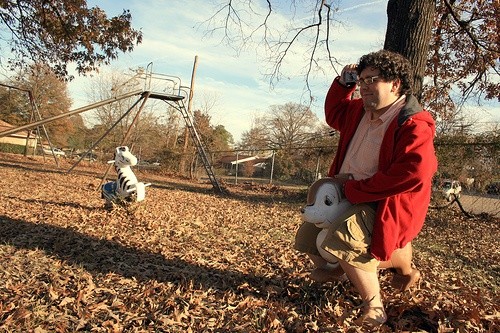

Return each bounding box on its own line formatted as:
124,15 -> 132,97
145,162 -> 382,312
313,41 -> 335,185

77,151 -> 97,160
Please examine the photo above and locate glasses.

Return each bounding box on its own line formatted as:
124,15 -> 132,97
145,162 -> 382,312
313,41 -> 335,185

356,74 -> 384,86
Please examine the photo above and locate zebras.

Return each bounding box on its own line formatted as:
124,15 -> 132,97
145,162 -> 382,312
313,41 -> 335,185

102,145 -> 138,205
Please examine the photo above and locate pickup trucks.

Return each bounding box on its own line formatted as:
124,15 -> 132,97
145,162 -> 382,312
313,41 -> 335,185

45,146 -> 66,156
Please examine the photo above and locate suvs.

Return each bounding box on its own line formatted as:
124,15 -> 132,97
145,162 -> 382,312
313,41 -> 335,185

440,179 -> 462,203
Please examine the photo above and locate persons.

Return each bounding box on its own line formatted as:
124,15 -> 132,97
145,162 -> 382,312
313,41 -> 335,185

291,49 -> 440,331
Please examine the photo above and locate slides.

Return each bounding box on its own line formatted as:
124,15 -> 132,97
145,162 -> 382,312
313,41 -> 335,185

0,88 -> 142,136
